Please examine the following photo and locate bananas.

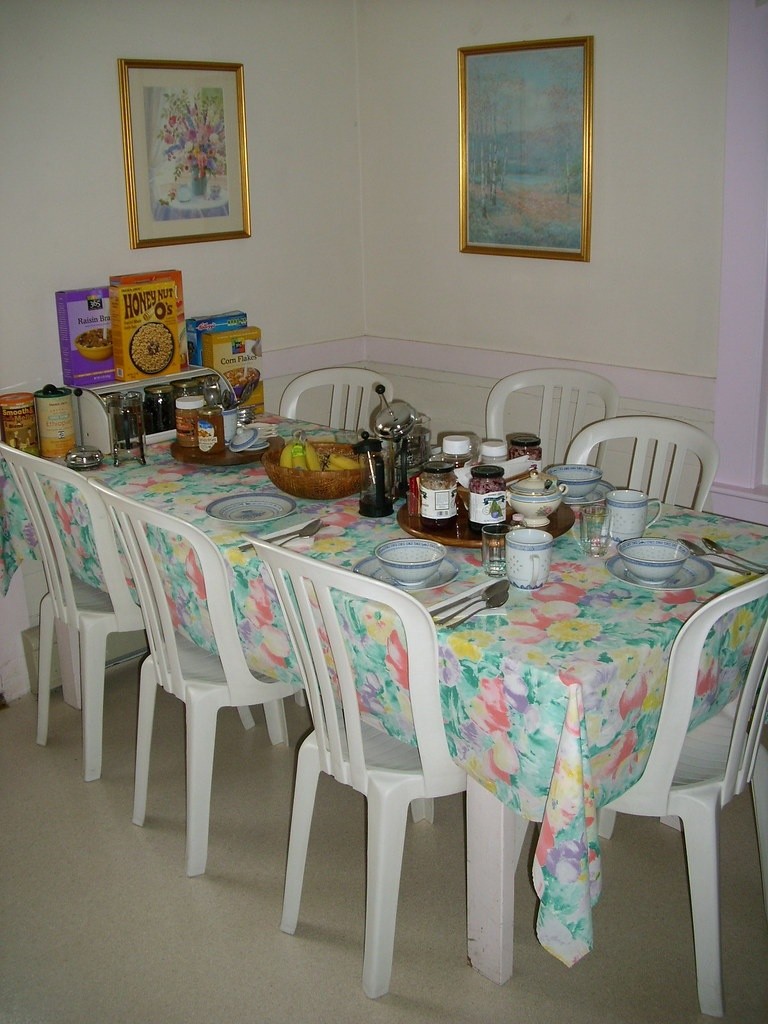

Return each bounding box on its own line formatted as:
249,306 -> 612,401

279,432 -> 361,471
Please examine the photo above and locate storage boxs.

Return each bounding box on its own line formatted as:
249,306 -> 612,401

201,326 -> 264,417
55,285 -> 115,386
186,308 -> 248,366
110,269 -> 190,370
109,279 -> 181,383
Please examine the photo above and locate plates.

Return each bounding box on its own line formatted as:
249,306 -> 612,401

353,556 -> 459,592
205,494 -> 296,524
129,321 -> 175,374
606,554 -> 715,591
562,481 -> 616,503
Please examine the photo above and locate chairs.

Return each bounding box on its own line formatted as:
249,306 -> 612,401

486,368 -> 620,469
279,366 -> 394,431
0,442 -> 257,782
239,531 -> 467,1001
563,414 -> 720,512
514,573 -> 768,1017
88,475 -> 306,877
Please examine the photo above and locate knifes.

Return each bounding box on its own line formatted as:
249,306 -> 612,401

429,588 -> 485,617
238,522 -> 330,550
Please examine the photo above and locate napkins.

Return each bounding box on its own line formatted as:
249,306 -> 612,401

240,518 -> 317,548
700,546 -> 743,577
427,577 -> 508,618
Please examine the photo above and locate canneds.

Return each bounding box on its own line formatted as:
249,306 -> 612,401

144,374 -> 223,454
419,435 -> 542,536
0,387 -> 78,459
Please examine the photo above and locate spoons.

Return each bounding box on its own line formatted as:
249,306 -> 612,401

677,538 -> 767,574
435,580 -> 510,629
277,519 -> 322,547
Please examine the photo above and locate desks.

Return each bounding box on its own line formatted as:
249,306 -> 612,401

0,410 -> 768,986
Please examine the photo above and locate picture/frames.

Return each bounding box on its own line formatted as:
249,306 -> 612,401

116,58 -> 252,251
456,35 -> 596,263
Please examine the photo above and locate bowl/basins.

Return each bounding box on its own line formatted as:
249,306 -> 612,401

75,327 -> 112,360
617,538 -> 691,584
546,465 -> 603,499
224,367 -> 260,398
376,541 -> 446,585
507,467 -> 568,528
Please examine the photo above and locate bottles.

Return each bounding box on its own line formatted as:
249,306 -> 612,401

375,386 -> 431,499
0,387 -> 76,460
144,375 -> 224,453
352,431 -> 394,516
511,514 -> 528,530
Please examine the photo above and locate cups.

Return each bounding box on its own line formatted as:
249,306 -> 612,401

579,506 -> 613,557
506,528 -> 554,591
606,491 -> 662,543
105,392 -> 146,462
482,525 -> 512,578
222,408 -> 237,446
